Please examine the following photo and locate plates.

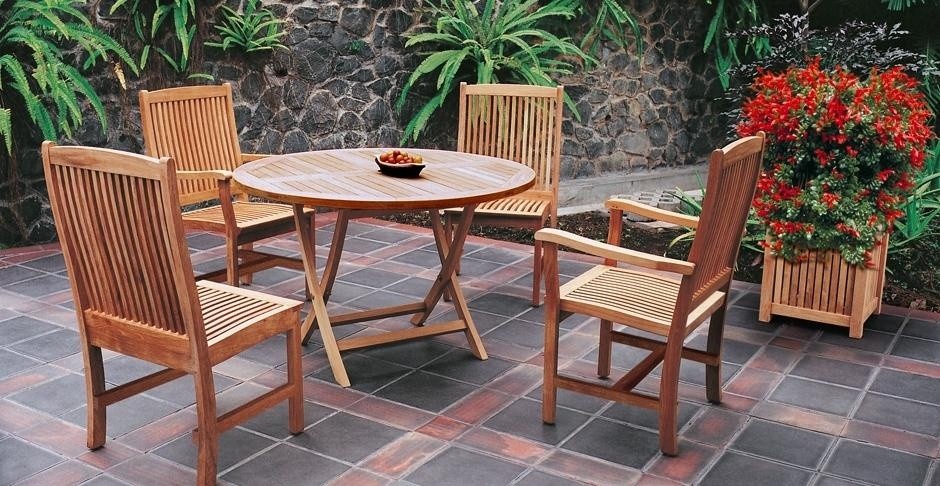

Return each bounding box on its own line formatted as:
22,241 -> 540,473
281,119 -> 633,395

373,152 -> 426,179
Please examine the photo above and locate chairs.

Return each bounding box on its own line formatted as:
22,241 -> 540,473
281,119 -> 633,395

534,130 -> 770,460
137,79 -> 317,302
440,79 -> 565,308
37,140 -> 306,485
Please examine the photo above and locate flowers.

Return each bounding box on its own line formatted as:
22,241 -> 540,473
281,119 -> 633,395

733,57 -> 934,268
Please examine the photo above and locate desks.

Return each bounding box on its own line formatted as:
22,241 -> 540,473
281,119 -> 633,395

230,144 -> 537,390
